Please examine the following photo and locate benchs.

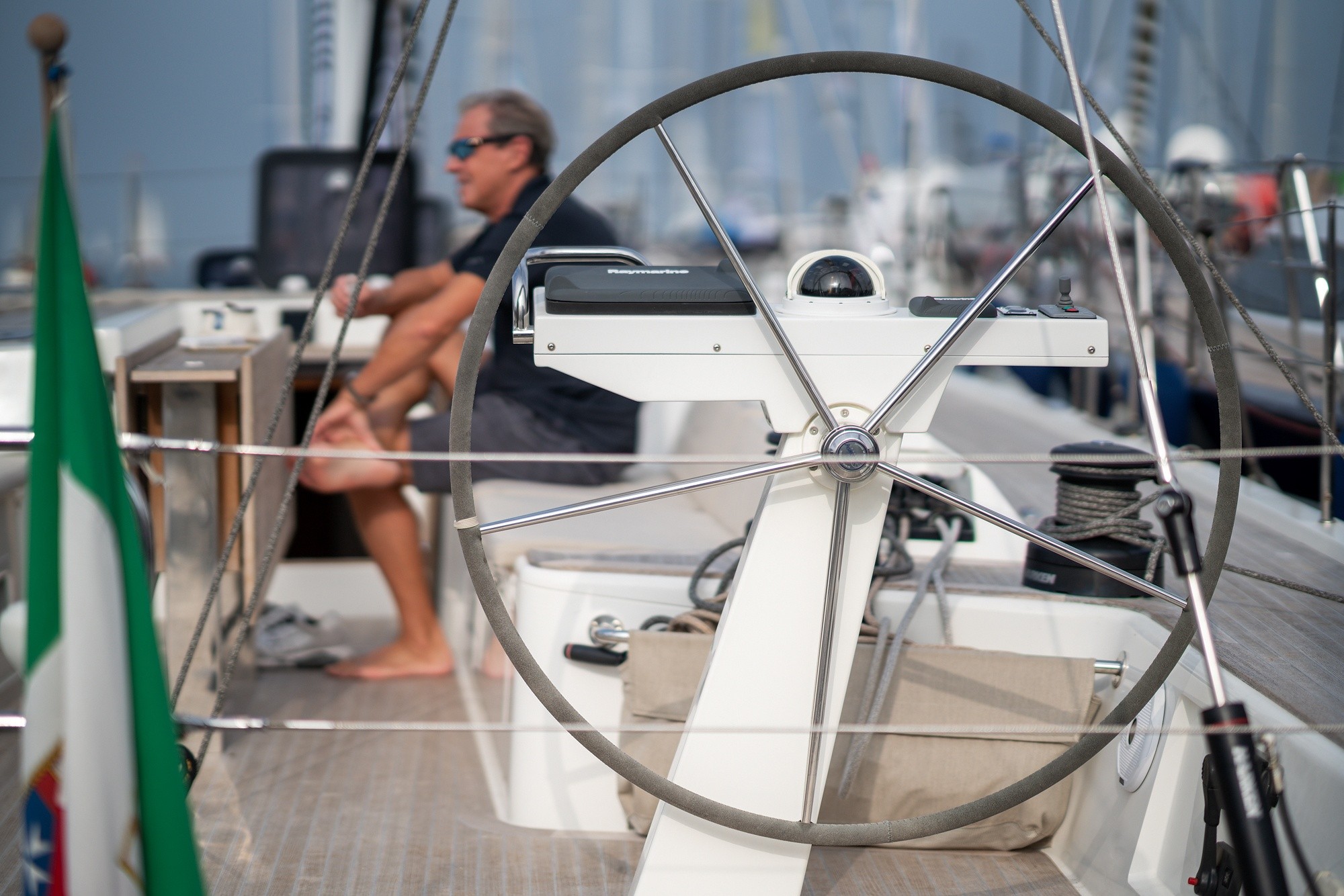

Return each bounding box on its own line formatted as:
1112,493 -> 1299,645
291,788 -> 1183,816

419,469 -> 723,830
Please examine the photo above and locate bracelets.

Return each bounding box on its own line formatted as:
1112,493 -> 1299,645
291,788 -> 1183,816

342,380 -> 376,409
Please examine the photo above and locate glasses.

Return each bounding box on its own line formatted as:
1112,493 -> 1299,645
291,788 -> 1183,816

445,131 -> 541,161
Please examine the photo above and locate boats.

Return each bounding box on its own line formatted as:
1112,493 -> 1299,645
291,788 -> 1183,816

882,157 -> 1344,433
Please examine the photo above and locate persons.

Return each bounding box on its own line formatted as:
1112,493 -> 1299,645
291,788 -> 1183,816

292,86 -> 640,681
